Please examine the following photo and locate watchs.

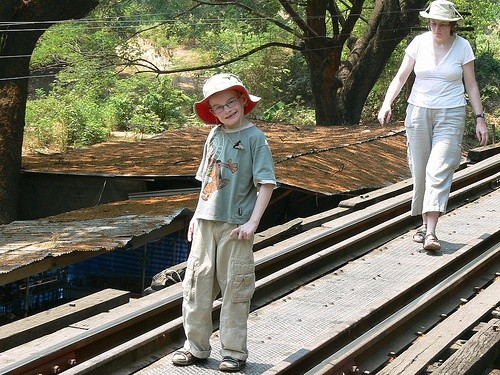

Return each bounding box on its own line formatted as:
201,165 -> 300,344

474,113 -> 484,120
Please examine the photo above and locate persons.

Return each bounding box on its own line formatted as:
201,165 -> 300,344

171,72 -> 275,374
377,1 -> 489,252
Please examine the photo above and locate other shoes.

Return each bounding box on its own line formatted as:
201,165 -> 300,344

423,232 -> 442,251
413,224 -> 428,242
219,356 -> 246,370
172,348 -> 206,365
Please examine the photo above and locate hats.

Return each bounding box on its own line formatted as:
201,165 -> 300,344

193,73 -> 261,126
419,0 -> 464,22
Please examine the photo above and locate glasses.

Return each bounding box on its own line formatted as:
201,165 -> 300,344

211,93 -> 242,114
